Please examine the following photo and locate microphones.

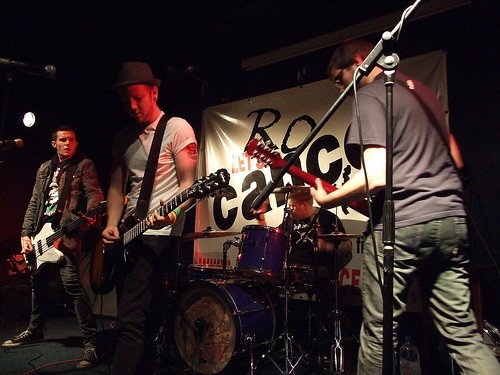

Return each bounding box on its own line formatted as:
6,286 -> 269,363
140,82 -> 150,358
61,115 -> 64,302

0,58 -> 56,78
0,139 -> 24,148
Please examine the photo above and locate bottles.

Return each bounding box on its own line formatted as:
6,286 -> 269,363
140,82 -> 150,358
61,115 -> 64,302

399,336 -> 421,375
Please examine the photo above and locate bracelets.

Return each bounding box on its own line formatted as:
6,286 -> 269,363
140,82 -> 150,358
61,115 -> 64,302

170,211 -> 177,226
173,208 -> 182,219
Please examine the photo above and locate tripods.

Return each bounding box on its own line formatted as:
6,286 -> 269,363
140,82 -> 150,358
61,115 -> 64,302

247,193 -> 362,375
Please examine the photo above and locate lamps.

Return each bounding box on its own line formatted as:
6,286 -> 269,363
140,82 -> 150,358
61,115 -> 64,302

12,111 -> 35,128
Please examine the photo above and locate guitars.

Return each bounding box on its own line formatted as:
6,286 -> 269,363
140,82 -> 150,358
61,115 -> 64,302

23,196 -> 127,275
244,138 -> 372,220
89,168 -> 230,295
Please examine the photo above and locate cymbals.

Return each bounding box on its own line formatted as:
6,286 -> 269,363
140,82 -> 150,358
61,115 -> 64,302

256,185 -> 311,192
181,230 -> 241,239
316,232 -> 365,244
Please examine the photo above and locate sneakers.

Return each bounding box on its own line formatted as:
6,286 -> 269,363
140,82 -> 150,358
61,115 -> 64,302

1,326 -> 45,348
76,341 -> 102,369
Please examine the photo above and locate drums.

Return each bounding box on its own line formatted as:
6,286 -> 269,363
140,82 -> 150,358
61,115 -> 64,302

172,278 -> 276,374
234,225 -> 291,281
187,264 -> 236,282
286,262 -> 314,293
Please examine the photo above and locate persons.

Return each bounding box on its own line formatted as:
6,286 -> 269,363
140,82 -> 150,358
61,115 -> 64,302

253,189 -> 347,266
2,125 -> 106,370
310,38 -> 500,375
101,62 -> 199,375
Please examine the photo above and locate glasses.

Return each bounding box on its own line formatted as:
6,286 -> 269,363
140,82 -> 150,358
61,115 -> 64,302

334,68 -> 345,89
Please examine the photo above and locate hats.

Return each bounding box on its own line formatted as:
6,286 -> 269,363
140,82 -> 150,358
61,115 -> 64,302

112,60 -> 161,88
